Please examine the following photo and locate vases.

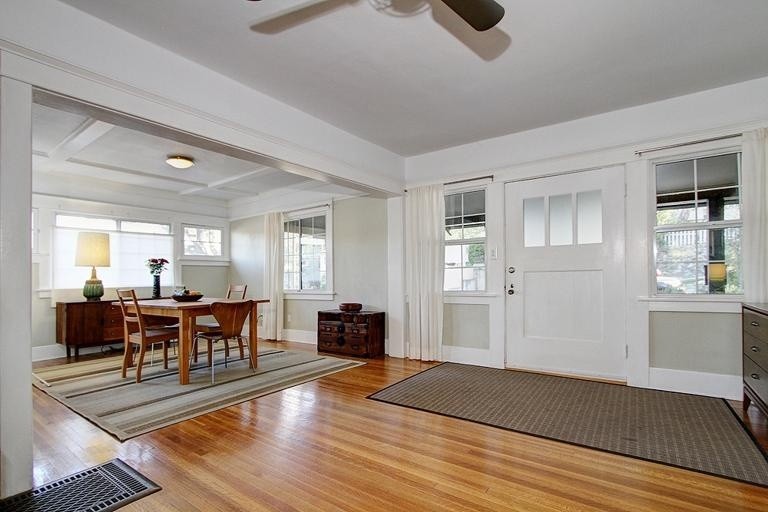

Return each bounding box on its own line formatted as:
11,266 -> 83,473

153,275 -> 160,297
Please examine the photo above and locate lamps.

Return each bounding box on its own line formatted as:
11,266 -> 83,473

166,156 -> 194,169
75,231 -> 111,298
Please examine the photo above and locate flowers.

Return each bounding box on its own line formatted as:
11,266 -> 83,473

144,258 -> 170,275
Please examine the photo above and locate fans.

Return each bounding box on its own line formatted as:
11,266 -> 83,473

245,0 -> 505,32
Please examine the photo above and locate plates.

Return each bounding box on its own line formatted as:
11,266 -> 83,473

171,294 -> 203,302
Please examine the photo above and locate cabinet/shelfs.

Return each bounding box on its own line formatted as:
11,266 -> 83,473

317,309 -> 385,359
742,301 -> 768,421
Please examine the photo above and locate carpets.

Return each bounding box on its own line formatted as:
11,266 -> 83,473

32,337 -> 368,443
0,457 -> 162,512
365,362 -> 768,489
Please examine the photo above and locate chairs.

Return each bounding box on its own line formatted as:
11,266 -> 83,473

115,280 -> 270,384
